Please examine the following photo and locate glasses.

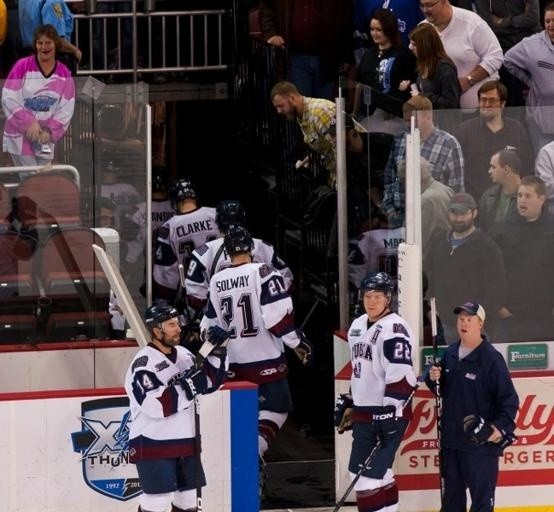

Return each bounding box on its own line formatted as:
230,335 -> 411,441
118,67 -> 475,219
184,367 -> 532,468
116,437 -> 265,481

479,98 -> 503,105
419,0 -> 440,8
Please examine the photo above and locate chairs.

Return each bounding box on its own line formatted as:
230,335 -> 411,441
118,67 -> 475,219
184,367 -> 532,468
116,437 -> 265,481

0,174 -> 113,341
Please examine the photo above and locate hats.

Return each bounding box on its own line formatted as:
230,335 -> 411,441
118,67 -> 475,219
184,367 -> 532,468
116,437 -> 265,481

323,113 -> 355,136
448,192 -> 478,213
452,302 -> 486,321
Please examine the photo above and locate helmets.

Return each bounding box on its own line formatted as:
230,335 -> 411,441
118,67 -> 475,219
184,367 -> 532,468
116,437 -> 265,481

224,224 -> 253,255
169,177 -> 197,202
215,198 -> 247,225
143,300 -> 182,326
360,271 -> 395,292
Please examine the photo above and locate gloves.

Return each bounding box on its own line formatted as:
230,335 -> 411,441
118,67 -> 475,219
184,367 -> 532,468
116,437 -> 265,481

463,413 -> 512,450
292,328 -> 315,367
372,404 -> 400,450
201,324 -> 230,349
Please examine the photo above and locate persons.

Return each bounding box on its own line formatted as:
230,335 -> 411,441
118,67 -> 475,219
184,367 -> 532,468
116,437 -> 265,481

124,299 -> 229,512
197,224 -> 314,478
424,301 -> 519,512
334,272 -> 418,512
